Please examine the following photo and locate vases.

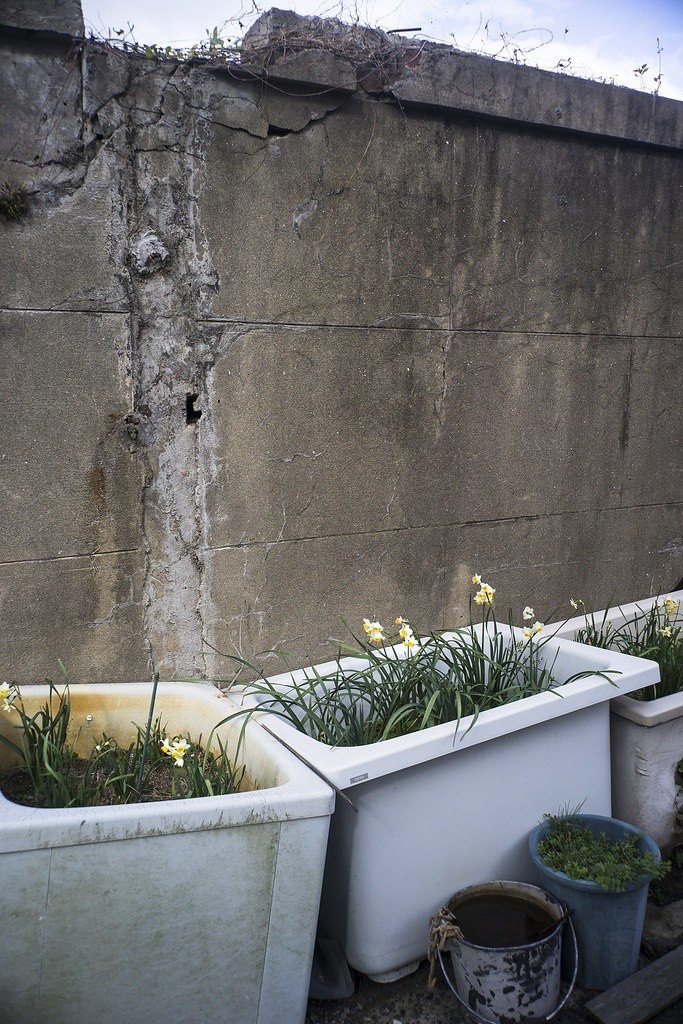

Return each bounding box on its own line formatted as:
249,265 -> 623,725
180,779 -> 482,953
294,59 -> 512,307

222,621 -> 661,984
531,590 -> 683,849
0,682 -> 335,1024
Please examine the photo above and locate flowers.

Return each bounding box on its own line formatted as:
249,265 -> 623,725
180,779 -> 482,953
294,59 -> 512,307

570,584 -> 683,701
0,657 -> 253,809
168,573 -> 623,776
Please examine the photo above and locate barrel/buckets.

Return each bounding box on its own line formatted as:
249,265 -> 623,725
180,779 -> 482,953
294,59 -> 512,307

436,880 -> 578,1024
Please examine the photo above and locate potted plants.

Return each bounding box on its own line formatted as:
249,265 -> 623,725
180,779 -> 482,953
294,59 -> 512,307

529,796 -> 671,990
